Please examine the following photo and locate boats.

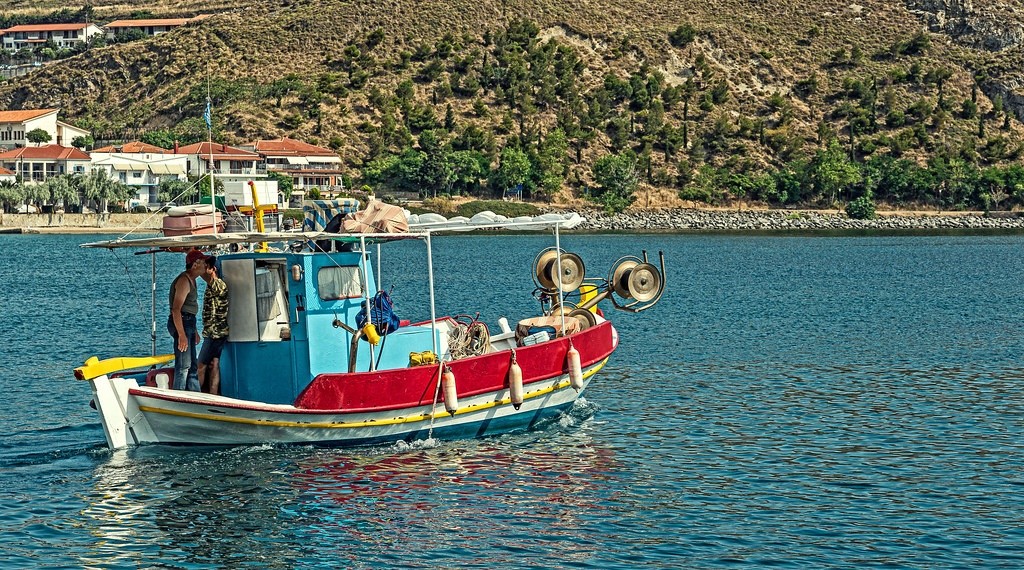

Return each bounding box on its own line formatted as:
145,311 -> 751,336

71,56 -> 669,451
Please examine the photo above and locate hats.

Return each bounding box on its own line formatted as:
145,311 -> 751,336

186,251 -> 210,264
206,256 -> 222,278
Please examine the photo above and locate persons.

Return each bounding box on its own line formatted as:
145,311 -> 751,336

166,250 -> 207,393
196,255 -> 229,394
293,217 -> 296,229
